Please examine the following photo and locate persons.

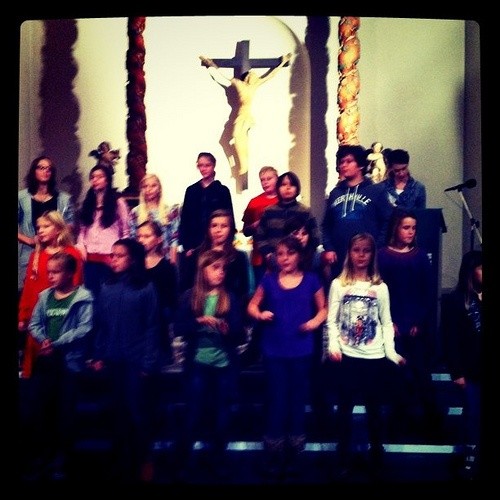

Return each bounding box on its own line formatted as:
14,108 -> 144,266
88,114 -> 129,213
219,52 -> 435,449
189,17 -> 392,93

189,208 -> 257,323
17,155 -> 76,305
127,219 -> 182,317
326,230 -> 406,467
241,165 -> 283,247
373,146 -> 427,242
377,210 -> 437,411
85,236 -> 166,466
268,213 -> 326,305
196,52 -> 296,191
73,163 -> 133,288
245,233 -> 329,460
322,143 -> 388,283
444,248 -> 483,403
130,173 -> 181,236
256,171 -> 320,278
170,248 -> 242,460
24,252 -> 93,458
19,209 -> 83,383
180,152 -> 237,289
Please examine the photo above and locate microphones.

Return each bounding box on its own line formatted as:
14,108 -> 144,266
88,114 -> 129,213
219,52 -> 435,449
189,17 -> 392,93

444,179 -> 477,192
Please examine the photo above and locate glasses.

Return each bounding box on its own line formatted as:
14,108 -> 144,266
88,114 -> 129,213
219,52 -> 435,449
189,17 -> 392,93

340,159 -> 357,164
90,174 -> 107,179
110,253 -> 129,259
35,165 -> 53,171
